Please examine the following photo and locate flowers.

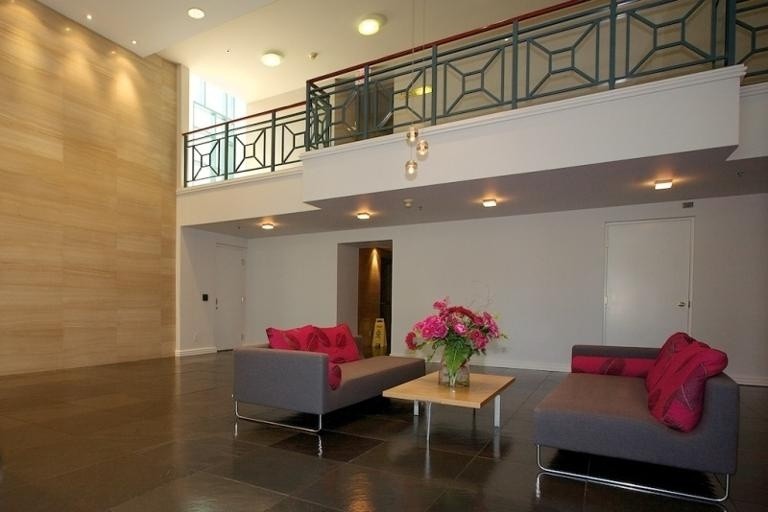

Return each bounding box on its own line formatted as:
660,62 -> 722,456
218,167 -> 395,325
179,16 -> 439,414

403,297 -> 511,378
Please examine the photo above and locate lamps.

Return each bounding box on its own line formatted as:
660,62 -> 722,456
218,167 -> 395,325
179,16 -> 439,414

405,0 -> 429,175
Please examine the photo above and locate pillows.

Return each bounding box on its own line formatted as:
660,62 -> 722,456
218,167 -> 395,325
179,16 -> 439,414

648,348 -> 729,433
572,355 -> 655,377
266,325 -> 344,364
644,332 -> 697,393
327,361 -> 342,390
320,323 -> 363,364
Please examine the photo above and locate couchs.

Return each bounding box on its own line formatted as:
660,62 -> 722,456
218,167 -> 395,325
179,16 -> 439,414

531,343 -> 740,512
232,343 -> 426,458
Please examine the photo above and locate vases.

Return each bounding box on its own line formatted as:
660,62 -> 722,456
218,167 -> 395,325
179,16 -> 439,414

438,363 -> 469,387
